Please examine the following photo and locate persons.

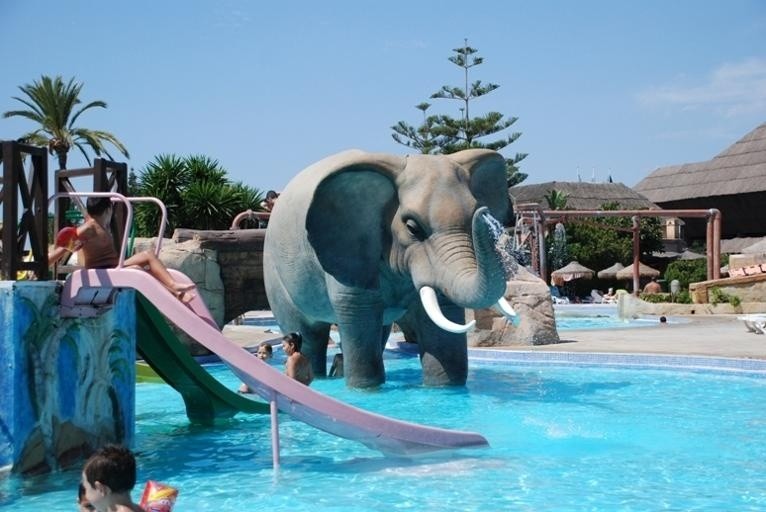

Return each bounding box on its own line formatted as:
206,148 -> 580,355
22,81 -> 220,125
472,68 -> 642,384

281,331 -> 314,386
327,343 -> 344,379
671,275 -> 682,304
573,296 -> 583,304
237,342 -> 272,394
598,287 -> 619,300
76,481 -> 98,512
257,190 -> 280,228
26,195 -> 197,306
82,441 -> 145,512
643,276 -> 662,294
658,316 -> 668,326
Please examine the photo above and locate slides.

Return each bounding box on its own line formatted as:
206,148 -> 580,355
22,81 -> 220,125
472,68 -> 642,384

136,292 -> 282,418
54,267 -> 488,467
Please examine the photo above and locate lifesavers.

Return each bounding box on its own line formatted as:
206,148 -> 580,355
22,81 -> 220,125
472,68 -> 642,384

140,480 -> 179,512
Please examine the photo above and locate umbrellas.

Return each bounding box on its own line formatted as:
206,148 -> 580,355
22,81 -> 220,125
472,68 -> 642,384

598,262 -> 625,279
550,260 -> 595,285
617,261 -> 661,279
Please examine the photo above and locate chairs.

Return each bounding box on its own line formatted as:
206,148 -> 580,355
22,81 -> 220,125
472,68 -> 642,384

738,317 -> 766,334
549,287 -> 570,305
591,289 -> 618,303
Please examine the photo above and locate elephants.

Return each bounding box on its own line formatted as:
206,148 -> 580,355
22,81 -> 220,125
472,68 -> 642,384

260,145 -> 513,390
